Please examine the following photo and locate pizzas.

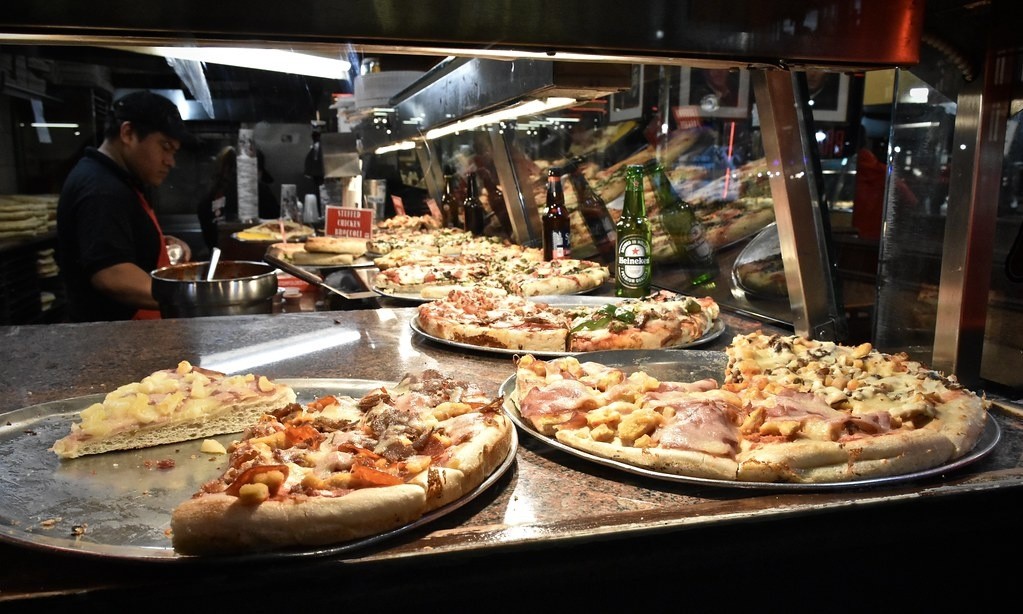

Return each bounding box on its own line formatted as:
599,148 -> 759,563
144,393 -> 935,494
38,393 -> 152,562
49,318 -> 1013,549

511,331 -> 993,482
234,215 -> 719,350
171,369 -> 513,556
51,361 -> 297,459
480,124 -> 790,293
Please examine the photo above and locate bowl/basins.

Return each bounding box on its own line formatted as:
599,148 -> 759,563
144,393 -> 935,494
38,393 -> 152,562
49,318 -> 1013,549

230,232 -> 317,258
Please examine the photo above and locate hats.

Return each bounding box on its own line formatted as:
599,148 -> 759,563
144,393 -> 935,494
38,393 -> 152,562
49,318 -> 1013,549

104,90 -> 199,140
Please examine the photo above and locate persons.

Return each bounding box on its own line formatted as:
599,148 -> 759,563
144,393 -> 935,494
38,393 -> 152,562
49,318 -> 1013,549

57,90 -> 191,321
800,71 -> 834,109
694,70 -> 736,105
854,137 -> 916,236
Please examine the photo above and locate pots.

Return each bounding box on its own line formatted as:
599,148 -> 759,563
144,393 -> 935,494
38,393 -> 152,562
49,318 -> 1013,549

150,260 -> 278,319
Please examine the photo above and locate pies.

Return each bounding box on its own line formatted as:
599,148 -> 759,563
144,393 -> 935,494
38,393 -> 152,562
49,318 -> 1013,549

0,193 -> 59,312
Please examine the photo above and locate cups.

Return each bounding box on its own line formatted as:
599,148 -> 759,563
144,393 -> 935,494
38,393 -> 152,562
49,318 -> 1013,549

236,129 -> 319,225
362,179 -> 386,224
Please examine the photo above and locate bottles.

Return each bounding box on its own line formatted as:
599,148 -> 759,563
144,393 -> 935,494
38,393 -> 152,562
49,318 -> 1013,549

478,166 -> 514,236
542,170 -> 572,262
442,173 -> 458,229
615,164 -> 651,299
463,173 -> 484,236
645,159 -> 720,285
564,163 -> 616,264
